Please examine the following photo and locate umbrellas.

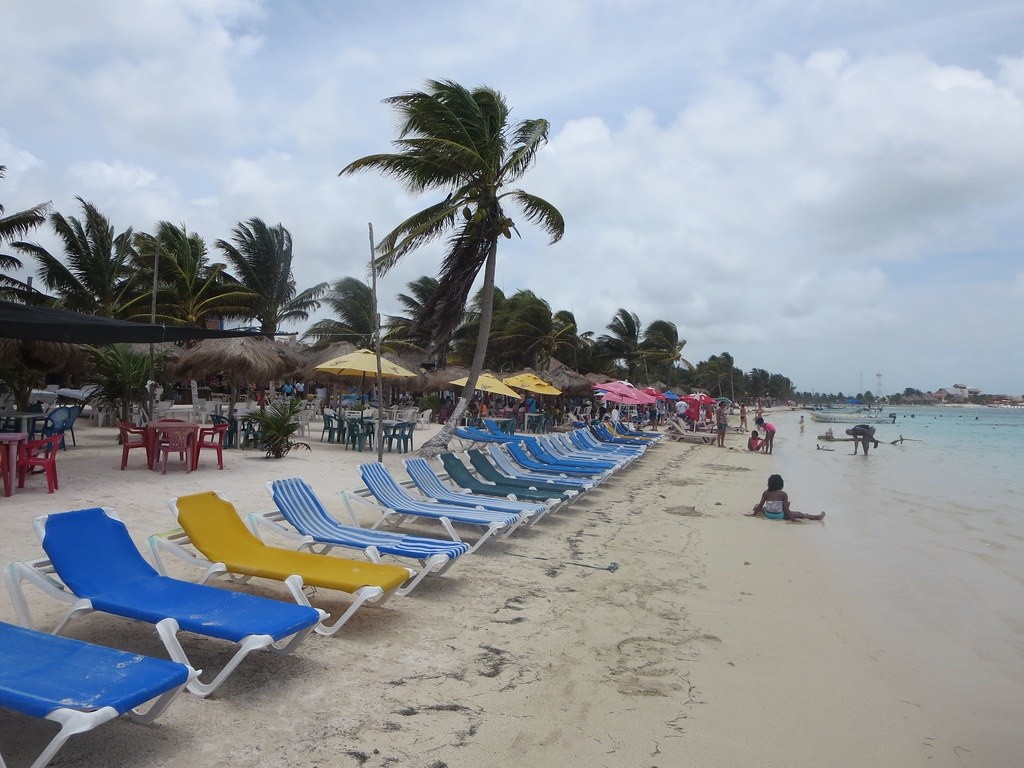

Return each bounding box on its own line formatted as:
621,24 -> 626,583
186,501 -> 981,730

449,372 -> 522,417
436,366 -> 613,397
127,334 -> 428,445
503,373 -> 562,395
593,381 -> 718,444
314,348 -> 417,428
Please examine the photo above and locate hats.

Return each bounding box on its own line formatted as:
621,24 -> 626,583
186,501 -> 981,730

531,394 -> 534,397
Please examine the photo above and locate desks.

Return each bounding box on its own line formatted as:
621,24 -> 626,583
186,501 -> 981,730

146,422 -> 200,475
0,433 -> 29,497
227,412 -> 255,449
477,418 -> 512,431
363,419 -> 398,453
0,412 -> 49,444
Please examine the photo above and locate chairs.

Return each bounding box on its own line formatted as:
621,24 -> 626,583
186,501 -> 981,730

0,395 -> 749,768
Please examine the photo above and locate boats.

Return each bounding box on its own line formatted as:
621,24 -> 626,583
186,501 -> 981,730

808,411 -> 896,424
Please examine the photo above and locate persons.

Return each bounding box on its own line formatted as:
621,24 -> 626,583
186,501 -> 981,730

471,395 -> 577,417
703,403 -> 715,420
317,384 -> 327,399
295,380 -> 304,400
740,404 -> 748,432
282,381 -> 294,397
800,416 -> 804,422
748,417 -> 776,454
599,396 -> 607,418
716,401 -> 728,447
756,406 -> 764,430
675,397 -> 688,417
349,383 -> 356,394
658,401 -> 666,417
649,403 -> 658,431
846,424 -> 876,455
444,396 -> 453,408
746,475 -> 826,523
